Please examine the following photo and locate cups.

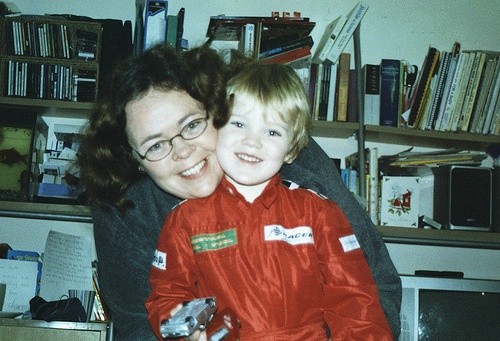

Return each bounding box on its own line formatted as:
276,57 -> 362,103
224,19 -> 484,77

61,290 -> 96,323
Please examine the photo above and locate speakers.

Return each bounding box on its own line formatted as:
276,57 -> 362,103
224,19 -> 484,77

433,164 -> 493,231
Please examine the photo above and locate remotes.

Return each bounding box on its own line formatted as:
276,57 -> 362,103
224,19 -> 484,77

415,270 -> 464,278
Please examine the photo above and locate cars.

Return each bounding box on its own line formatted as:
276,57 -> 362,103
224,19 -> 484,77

158,296 -> 218,340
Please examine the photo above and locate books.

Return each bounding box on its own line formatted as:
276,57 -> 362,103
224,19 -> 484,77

8,61 -> 72,100
204,2 -> 369,122
9,21 -> 69,59
341,146 -> 378,226
363,41 -> 500,135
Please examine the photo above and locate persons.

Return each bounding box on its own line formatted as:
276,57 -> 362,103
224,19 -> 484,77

145,60 -> 394,341
77,45 -> 402,341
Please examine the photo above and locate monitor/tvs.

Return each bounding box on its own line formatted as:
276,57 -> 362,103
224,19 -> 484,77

398,275 -> 500,341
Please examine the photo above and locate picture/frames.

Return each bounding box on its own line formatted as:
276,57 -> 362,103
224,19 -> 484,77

28,111 -> 94,200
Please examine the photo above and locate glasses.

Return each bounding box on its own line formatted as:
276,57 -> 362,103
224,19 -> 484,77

127,107 -> 209,162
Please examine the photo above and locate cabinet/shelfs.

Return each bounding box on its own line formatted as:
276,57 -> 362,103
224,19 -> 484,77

0,15 -> 500,341
